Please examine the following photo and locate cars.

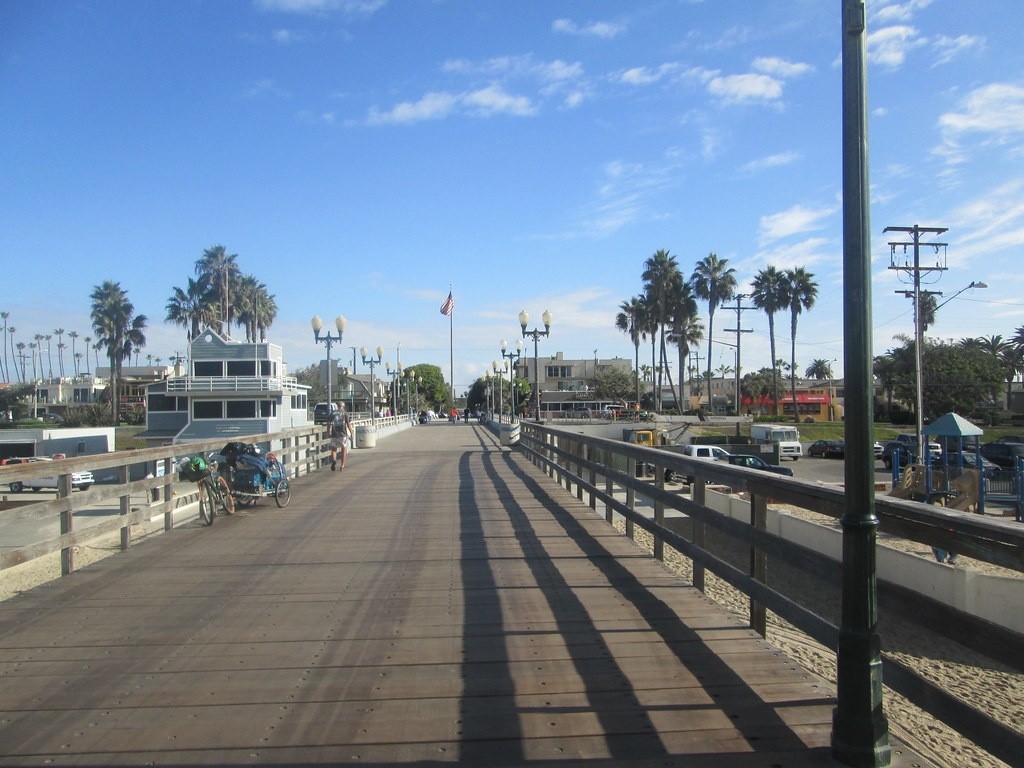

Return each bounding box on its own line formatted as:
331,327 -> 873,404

808,440 -> 845,459
559,407 -> 592,418
874,435 -> 1024,479
3,457 -> 95,493
43,414 -> 64,422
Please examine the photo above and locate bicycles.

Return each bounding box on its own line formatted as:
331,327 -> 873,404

180,454 -> 236,526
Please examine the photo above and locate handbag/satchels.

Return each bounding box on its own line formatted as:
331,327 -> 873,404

457,415 -> 460,420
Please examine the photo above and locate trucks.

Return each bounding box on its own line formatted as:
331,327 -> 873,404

750,424 -> 803,461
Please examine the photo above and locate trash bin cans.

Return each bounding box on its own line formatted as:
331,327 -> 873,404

419,412 -> 431,424
499,423 -> 520,446
356,426 -> 375,448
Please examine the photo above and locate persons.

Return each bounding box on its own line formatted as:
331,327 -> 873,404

379,410 -> 383,417
145,472 -> 154,503
327,401 -> 354,470
451,407 -> 458,424
476,409 -> 482,423
610,409 -> 623,417
386,409 -> 391,416
464,409 -> 469,423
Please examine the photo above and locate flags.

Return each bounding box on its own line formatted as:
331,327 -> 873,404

440,293 -> 455,316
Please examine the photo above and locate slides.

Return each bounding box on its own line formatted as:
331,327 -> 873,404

944,494 -> 977,511
888,487 -> 911,499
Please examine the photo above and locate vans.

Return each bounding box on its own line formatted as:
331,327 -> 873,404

313,403 -> 339,425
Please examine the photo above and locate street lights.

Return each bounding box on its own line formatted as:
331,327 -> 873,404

492,360 -> 509,423
482,370 -> 499,420
730,348 -> 737,412
500,339 -> 523,423
311,315 -> 347,437
828,359 -> 837,421
519,309 -> 552,424
35,349 -> 48,417
916,282 -> 988,466
385,361 -> 403,420
400,370 -> 422,417
360,346 -> 384,425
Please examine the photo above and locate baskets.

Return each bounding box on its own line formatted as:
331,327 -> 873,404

180,456 -> 212,483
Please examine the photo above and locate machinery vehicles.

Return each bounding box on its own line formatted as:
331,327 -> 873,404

622,421 -> 692,477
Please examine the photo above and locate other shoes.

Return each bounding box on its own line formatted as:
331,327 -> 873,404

331,460 -> 338,470
341,467 -> 346,471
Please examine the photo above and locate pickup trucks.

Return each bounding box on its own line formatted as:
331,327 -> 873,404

644,446 -> 794,491
